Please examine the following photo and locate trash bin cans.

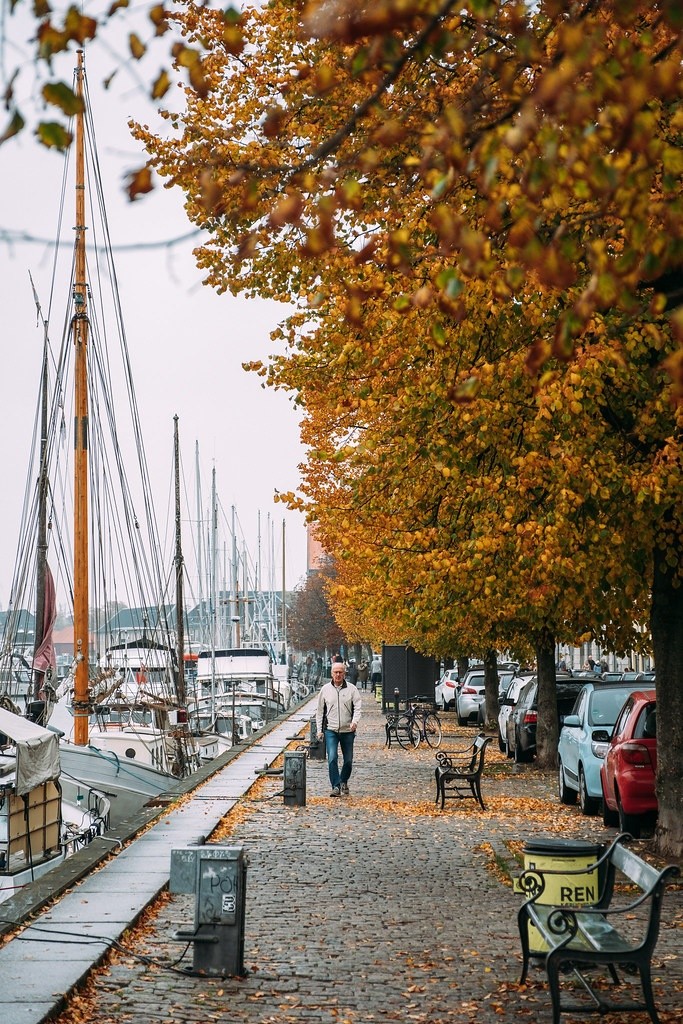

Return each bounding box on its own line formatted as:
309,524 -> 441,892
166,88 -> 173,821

523,839 -> 605,955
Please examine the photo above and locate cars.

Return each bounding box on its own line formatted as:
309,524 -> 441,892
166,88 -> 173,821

455,669 -> 514,727
503,673 -> 606,765
591,687 -> 657,835
498,660 -> 657,683
434,668 -> 458,712
453,668 -> 484,712
497,673 -> 569,751
555,682 -> 657,815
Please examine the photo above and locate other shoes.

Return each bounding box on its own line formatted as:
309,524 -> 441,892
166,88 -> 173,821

362,688 -> 366,693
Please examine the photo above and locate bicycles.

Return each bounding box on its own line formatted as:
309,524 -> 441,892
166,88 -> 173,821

394,696 -> 442,750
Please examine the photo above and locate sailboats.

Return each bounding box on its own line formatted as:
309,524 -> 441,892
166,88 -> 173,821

1,30 -> 304,902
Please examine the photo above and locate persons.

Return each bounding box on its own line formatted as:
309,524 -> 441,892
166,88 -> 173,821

556,655 -> 636,673
317,663 -> 362,798
279,652 -> 382,692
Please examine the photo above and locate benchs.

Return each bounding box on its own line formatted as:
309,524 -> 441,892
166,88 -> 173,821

517,832 -> 681,1024
384,704 -> 420,749
435,733 -> 493,810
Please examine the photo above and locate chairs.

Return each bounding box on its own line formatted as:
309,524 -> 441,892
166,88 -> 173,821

641,712 -> 656,738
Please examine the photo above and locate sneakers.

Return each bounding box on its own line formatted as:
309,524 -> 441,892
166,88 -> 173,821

329,787 -> 342,797
340,781 -> 349,794
